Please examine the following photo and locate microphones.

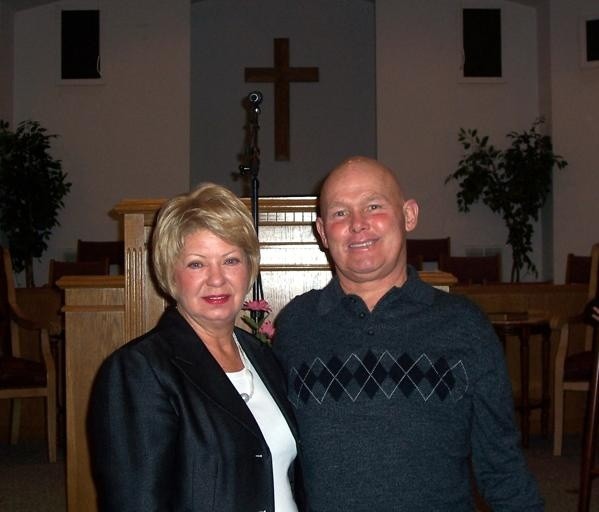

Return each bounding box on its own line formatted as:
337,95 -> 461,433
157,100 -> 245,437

249,91 -> 263,106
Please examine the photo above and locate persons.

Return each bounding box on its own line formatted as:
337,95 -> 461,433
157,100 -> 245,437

269,152 -> 547,512
86,183 -> 306,512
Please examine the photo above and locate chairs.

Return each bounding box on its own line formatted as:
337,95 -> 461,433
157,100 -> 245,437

0,239 -> 125,463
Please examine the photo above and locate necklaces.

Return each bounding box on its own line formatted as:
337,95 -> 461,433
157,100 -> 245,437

234,336 -> 255,402
